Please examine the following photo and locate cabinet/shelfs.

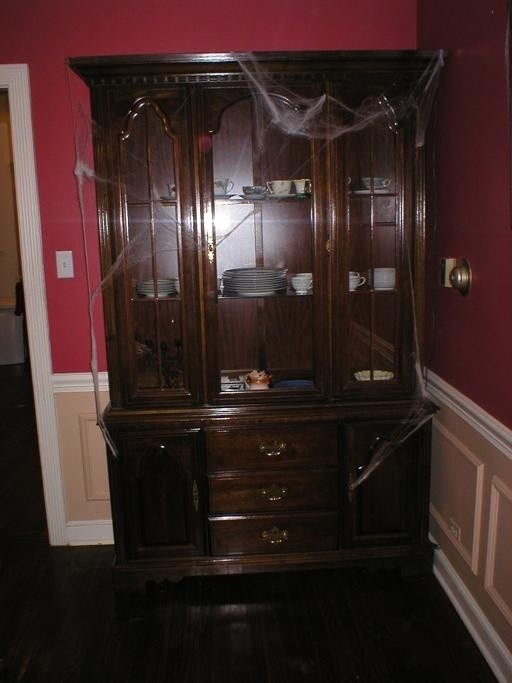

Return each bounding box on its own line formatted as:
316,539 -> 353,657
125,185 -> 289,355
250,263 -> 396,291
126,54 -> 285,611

69,50 -> 449,596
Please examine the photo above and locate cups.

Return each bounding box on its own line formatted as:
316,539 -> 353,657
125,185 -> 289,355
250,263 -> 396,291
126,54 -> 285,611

349,272 -> 366,292
362,176 -> 391,190
167,183 -> 176,196
213,177 -> 234,195
266,180 -> 293,195
291,273 -> 313,295
292,178 -> 311,193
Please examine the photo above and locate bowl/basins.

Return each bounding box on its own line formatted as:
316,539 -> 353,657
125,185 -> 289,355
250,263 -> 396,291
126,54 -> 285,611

243,185 -> 266,195
368,267 -> 396,291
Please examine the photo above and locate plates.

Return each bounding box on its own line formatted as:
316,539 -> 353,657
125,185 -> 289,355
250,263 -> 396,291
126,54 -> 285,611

354,369 -> 394,382
220,266 -> 288,295
239,194 -> 268,200
213,194 -> 237,199
137,277 -> 178,298
270,194 -> 296,199
353,190 -> 390,195
160,196 -> 177,200
